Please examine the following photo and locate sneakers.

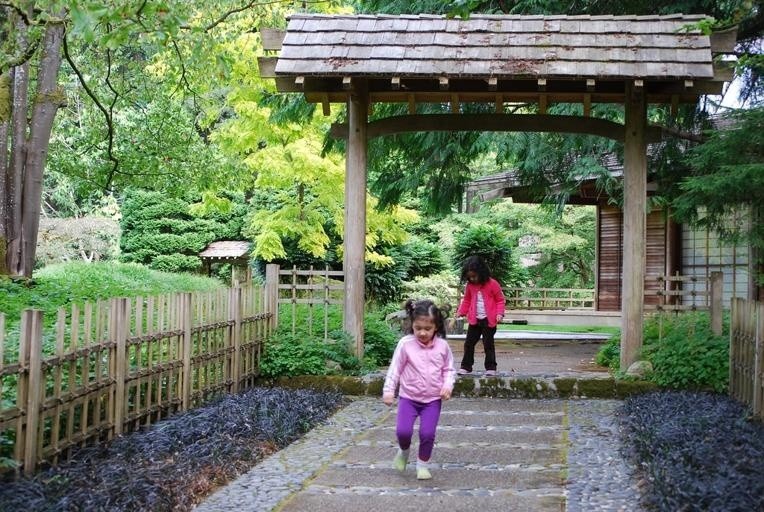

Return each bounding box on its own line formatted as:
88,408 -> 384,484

391,446 -> 409,475
456,368 -> 472,375
483,369 -> 496,377
416,461 -> 432,480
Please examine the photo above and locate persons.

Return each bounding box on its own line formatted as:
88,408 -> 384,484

382,297 -> 460,483
446,256 -> 508,377
